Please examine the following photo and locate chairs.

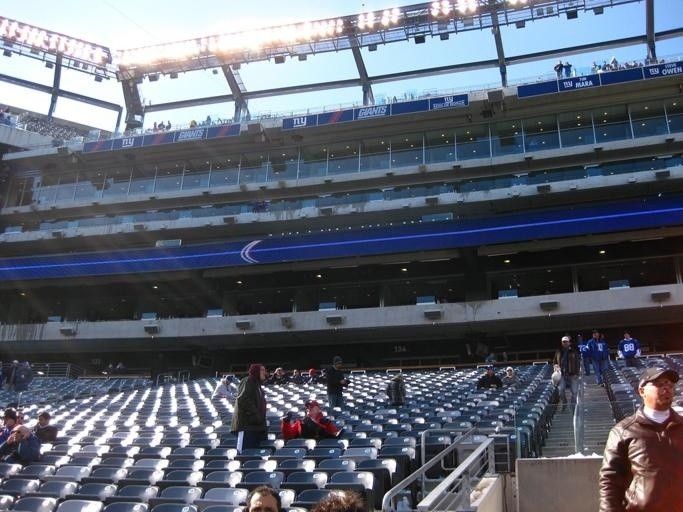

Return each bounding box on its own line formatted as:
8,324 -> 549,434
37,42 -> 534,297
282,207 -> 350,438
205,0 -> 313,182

603,354 -> 682,421
1,437 -> 417,512
0,363 -> 559,472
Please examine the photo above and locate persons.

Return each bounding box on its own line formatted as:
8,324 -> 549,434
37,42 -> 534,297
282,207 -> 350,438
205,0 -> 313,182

0,359 -> 58,467
152,114 -> 212,132
564,328 -> 642,386
212,367 -> 328,400
553,56 -> 665,79
326,354 -> 351,415
475,350 -> 522,391
599,366 -> 682,511
386,374 -> 407,407
278,400 -> 340,442
0,104 -> 12,126
245,484 -> 283,512
552,335 -> 582,416
228,363 -> 268,455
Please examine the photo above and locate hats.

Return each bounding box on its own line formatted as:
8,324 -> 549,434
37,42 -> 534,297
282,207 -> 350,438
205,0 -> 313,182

308,368 -> 316,375
333,356 -> 342,365
0,408 -> 17,419
226,374 -> 235,382
304,399 -> 320,409
561,336 -> 569,342
484,365 -> 495,371
637,366 -> 680,388
